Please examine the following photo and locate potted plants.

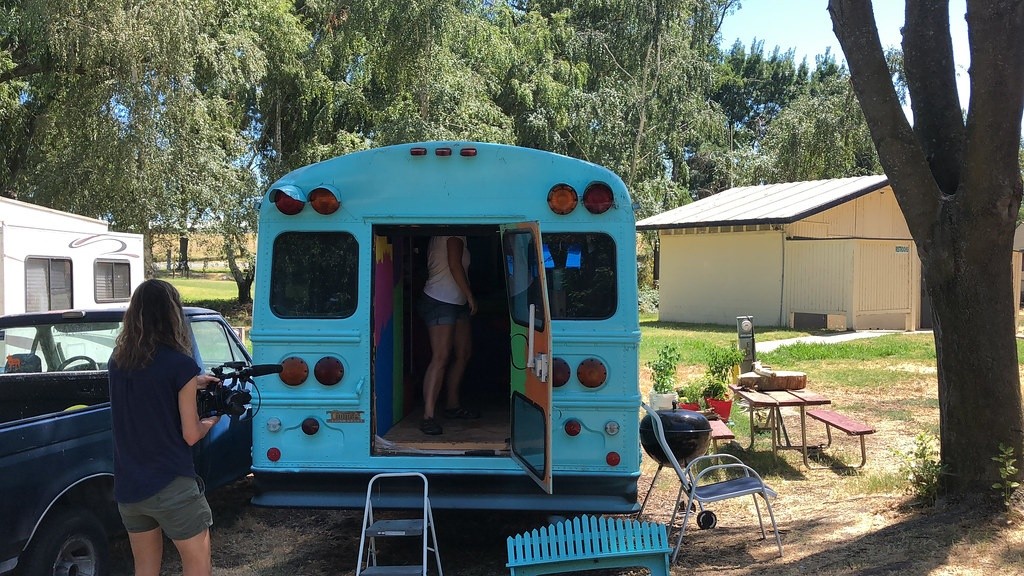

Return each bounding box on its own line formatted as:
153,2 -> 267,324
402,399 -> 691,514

678,387 -> 701,412
701,381 -> 733,423
645,344 -> 679,412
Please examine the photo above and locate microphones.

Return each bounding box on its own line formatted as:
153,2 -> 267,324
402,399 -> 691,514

229,364 -> 284,377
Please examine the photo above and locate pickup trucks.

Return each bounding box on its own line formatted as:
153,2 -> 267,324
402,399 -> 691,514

0,306 -> 251,576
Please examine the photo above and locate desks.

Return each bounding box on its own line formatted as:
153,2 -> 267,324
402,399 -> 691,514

708,383 -> 867,480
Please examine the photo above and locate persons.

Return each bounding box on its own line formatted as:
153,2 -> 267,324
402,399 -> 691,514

108,280 -> 221,576
419,236 -> 480,435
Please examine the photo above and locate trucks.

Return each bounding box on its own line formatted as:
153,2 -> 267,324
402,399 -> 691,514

243,139 -> 639,520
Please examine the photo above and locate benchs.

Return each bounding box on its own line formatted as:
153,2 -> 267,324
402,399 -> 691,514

807,409 -> 876,435
707,419 -> 735,439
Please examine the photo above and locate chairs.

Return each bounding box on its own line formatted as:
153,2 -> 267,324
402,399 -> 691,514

641,401 -> 784,563
4,354 -> 41,373
637,410 -> 718,531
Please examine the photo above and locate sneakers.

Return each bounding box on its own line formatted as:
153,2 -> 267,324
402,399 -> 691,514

419,419 -> 443,435
445,406 -> 481,419
